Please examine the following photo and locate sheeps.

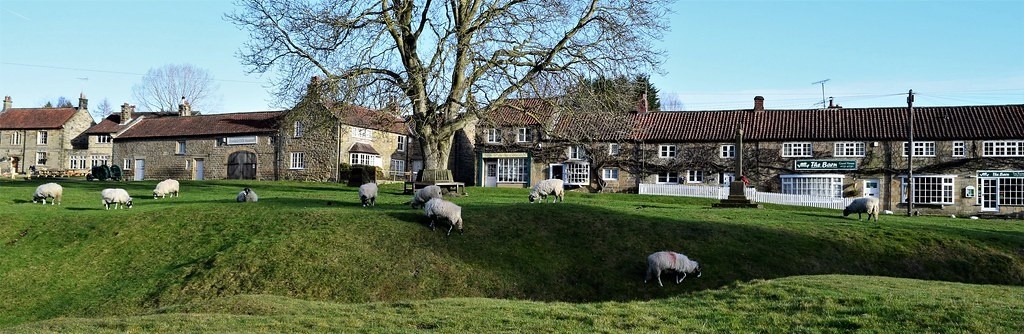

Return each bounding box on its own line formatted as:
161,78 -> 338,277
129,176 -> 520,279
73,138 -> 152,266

528,179 -> 565,204
101,188 -> 133,210
33,183 -> 63,205
412,185 -> 463,236
646,251 -> 702,287
843,196 -> 879,222
236,187 -> 258,202
153,178 -> 180,199
359,182 -> 378,208
